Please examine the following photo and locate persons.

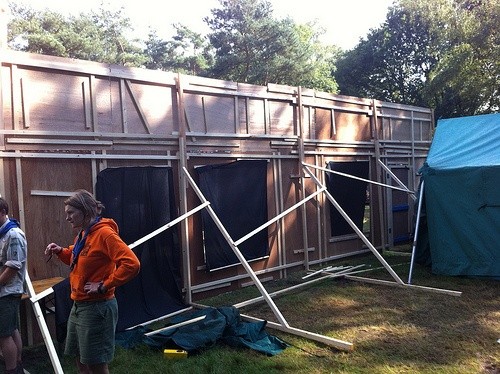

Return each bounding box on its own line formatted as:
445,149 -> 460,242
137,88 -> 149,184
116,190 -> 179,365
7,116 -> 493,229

45,191 -> 140,374
0,197 -> 27,374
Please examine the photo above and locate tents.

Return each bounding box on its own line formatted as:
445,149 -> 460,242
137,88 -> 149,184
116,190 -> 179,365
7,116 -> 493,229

408,113 -> 500,284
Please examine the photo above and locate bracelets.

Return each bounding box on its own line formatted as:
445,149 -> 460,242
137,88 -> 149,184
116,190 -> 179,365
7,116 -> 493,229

55,247 -> 63,255
97,281 -> 107,294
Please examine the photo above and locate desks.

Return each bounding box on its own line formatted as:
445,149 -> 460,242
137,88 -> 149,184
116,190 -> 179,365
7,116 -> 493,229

22,277 -> 70,345
